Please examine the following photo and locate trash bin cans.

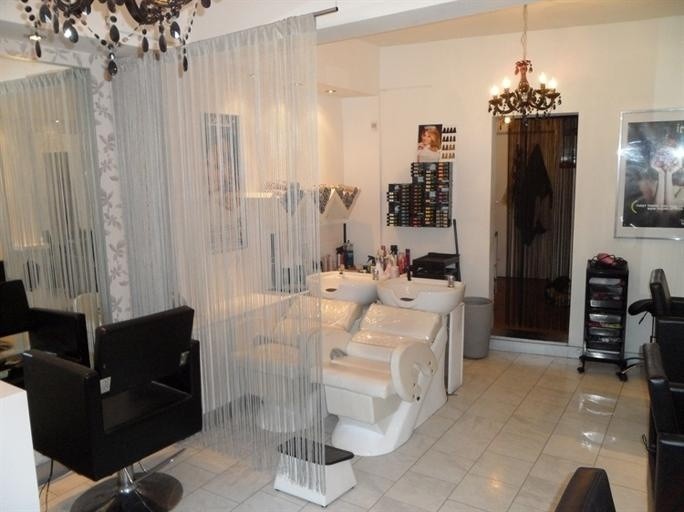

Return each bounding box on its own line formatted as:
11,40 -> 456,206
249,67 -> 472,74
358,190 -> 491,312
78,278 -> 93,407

463,297 -> 492,359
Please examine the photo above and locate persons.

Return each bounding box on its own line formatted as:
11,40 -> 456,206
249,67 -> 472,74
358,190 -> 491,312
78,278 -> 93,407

635,137 -> 684,228
417,124 -> 442,162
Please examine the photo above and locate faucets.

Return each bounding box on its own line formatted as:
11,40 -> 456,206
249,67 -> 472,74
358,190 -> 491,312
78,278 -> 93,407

446,274 -> 454,286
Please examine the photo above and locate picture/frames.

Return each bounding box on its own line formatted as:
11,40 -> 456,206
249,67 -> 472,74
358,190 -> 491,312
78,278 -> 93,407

613,108 -> 684,241
198,111 -> 246,253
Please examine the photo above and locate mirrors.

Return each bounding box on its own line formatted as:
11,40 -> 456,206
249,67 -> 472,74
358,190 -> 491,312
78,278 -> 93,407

0,55 -> 110,390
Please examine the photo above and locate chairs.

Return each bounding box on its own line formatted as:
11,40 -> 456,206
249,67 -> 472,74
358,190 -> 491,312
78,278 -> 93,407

0,261 -> 91,374
232,294 -> 448,457
21,293 -> 203,512
554,268 -> 684,512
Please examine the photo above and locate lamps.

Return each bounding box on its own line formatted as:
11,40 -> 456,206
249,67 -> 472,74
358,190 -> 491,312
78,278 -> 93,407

489,4 -> 561,130
20,0 -> 211,76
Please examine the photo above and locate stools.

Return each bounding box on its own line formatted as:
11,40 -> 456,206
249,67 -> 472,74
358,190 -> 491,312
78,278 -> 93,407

273,436 -> 357,507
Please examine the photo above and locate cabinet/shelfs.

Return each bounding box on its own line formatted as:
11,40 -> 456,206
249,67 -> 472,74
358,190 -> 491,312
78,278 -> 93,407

411,252 -> 462,282
576,259 -> 628,382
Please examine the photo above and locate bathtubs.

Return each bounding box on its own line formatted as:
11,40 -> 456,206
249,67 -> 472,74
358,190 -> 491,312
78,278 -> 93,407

193,291 -> 290,415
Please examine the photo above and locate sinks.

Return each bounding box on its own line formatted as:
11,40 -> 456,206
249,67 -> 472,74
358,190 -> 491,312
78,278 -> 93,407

376,276 -> 465,315
306,270 -> 378,304
